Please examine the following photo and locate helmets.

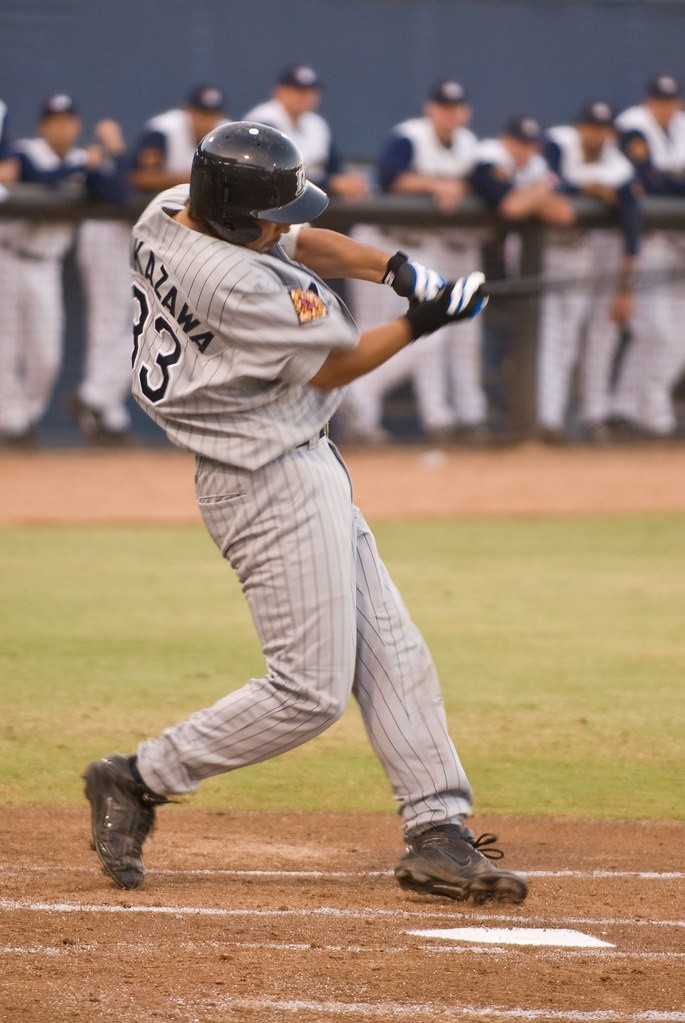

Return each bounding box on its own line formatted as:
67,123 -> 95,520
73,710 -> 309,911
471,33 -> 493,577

188,121 -> 329,245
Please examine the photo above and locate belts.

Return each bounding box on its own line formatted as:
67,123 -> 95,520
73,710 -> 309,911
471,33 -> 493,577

295,421 -> 329,448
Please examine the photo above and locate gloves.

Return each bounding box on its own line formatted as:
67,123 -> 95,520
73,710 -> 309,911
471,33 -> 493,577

407,271 -> 490,339
383,250 -> 445,303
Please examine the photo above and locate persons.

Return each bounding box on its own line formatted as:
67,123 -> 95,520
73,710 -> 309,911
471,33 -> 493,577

81,119 -> 529,906
241,60 -> 371,206
129,82 -> 239,192
75,130 -> 192,448
0,85 -> 128,451
331,73 -> 685,453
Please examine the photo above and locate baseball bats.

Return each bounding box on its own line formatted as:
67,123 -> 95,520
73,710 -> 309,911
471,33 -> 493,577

447,255 -> 685,298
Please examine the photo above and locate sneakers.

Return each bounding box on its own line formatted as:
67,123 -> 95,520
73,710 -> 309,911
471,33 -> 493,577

394,824 -> 528,905
80,753 -> 181,890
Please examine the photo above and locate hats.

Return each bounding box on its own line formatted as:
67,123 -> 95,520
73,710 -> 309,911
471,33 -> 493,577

280,65 -> 322,89
648,74 -> 679,99
38,93 -> 79,121
509,114 -> 544,141
429,80 -> 466,105
579,102 -> 614,123
189,85 -> 225,111
